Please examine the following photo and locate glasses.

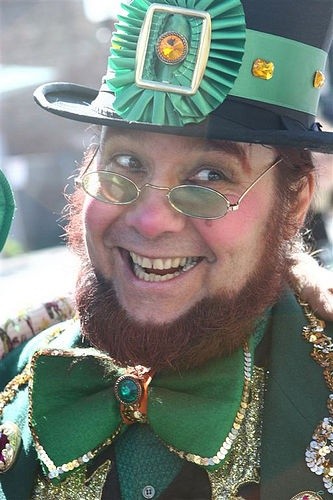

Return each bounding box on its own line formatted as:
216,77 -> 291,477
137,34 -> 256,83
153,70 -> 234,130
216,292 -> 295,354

74,147 -> 285,219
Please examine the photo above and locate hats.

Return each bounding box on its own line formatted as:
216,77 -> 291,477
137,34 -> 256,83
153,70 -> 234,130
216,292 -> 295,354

33,0 -> 333,155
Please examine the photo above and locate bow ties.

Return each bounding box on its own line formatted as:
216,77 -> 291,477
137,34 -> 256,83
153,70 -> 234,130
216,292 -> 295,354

29,336 -> 255,476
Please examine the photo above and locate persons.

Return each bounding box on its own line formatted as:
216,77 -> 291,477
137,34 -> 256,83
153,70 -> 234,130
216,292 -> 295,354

0,0 -> 333,500
